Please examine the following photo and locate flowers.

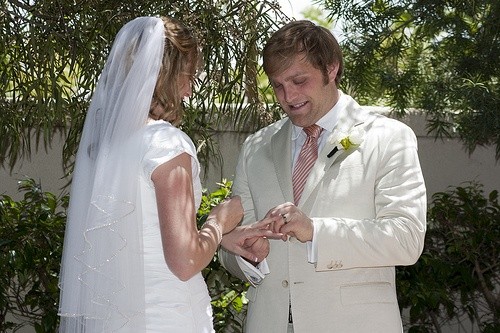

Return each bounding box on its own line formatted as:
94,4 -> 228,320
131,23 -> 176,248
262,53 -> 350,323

327,126 -> 366,158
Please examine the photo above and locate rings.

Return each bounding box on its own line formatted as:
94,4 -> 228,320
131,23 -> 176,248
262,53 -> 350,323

283,214 -> 288,224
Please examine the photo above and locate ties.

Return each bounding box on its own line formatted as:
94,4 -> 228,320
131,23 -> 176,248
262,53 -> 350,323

292,124 -> 323,209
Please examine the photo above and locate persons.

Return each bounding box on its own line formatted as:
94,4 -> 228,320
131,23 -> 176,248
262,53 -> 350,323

215,19 -> 428,333
54,15 -> 287,333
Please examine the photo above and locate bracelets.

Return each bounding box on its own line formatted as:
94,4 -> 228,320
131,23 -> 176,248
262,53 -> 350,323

200,221 -> 223,245
